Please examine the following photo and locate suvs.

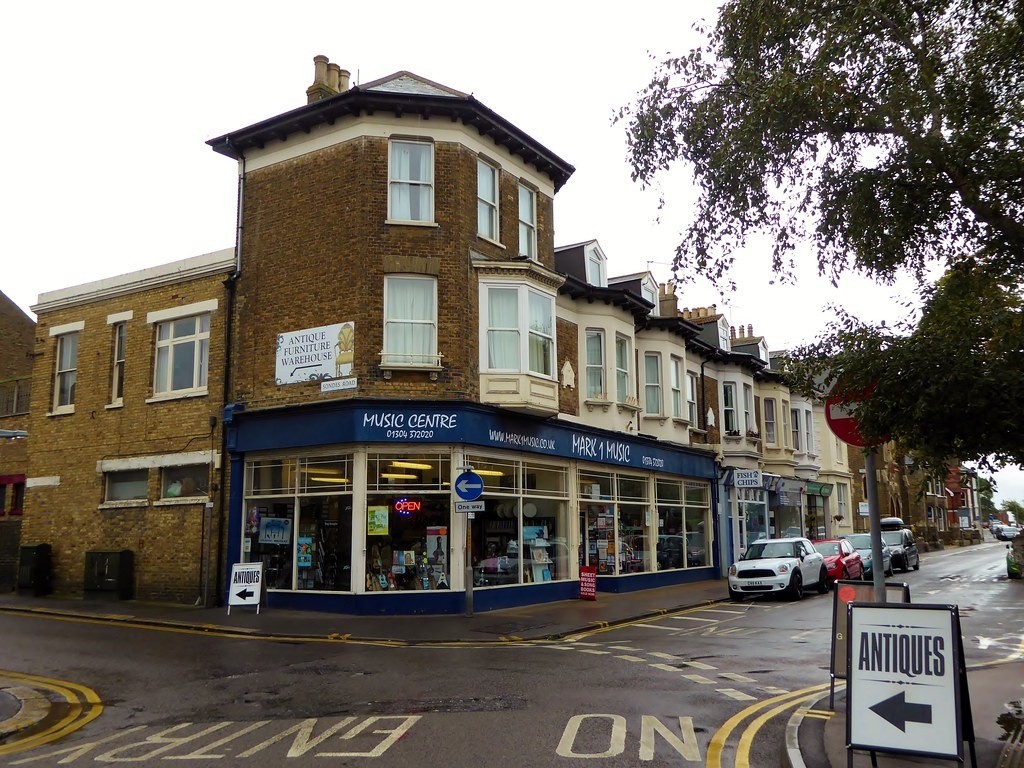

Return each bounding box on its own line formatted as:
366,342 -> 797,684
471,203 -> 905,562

729,536 -> 830,601
868,517 -> 921,572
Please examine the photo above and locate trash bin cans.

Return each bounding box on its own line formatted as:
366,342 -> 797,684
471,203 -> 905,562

17,542 -> 52,597
83,549 -> 135,602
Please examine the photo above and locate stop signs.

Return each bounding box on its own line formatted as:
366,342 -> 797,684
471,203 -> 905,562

826,367 -> 895,449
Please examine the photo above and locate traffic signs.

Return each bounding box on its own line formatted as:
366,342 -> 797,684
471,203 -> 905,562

852,608 -> 958,756
228,565 -> 262,604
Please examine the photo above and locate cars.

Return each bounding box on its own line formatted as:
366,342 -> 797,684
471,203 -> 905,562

1006,542 -> 1024,579
837,534 -> 894,579
988,522 -> 1009,535
810,539 -> 863,589
999,527 -> 1020,541
